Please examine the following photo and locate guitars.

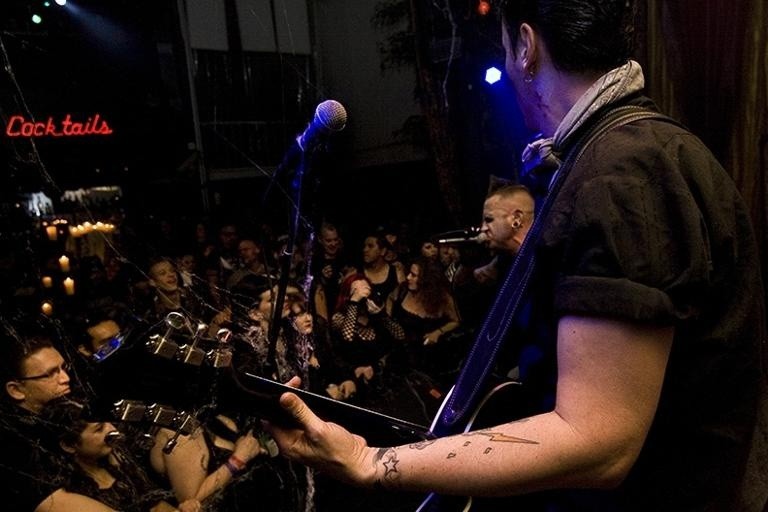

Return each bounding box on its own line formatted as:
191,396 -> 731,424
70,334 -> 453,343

79,312 -> 523,512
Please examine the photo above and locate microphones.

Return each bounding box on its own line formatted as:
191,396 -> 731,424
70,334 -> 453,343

275,100 -> 348,176
438,232 -> 487,245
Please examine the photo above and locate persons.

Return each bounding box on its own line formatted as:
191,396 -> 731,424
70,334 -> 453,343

259,0 -> 766,512
1,184 -> 537,512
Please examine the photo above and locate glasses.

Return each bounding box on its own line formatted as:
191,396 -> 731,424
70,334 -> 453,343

16,359 -> 71,381
